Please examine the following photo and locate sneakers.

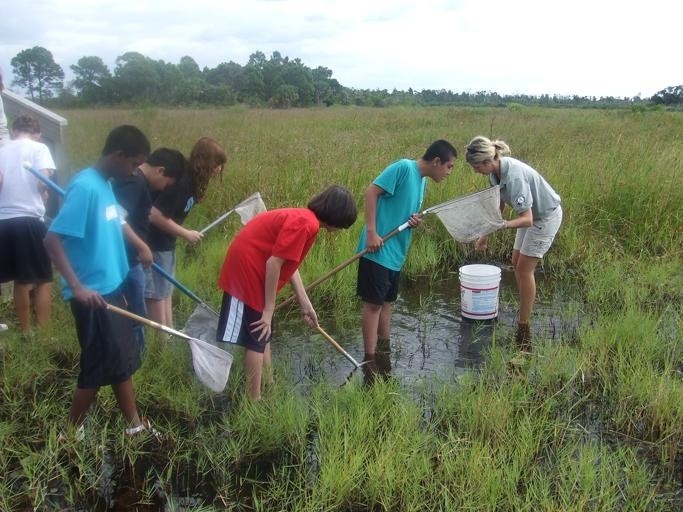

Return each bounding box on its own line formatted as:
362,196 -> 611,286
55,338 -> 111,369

54,425 -> 105,462
124,416 -> 179,457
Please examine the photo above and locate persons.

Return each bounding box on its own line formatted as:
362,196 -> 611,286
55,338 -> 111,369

41,123 -> 167,442
112,147 -> 185,357
215,184 -> 359,399
353,139 -> 458,356
464,137 -> 564,334
142,134 -> 230,328
0,115 -> 59,334
0,69 -> 8,332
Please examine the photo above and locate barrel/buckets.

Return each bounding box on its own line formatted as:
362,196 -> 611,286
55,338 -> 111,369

460,263 -> 501,320
